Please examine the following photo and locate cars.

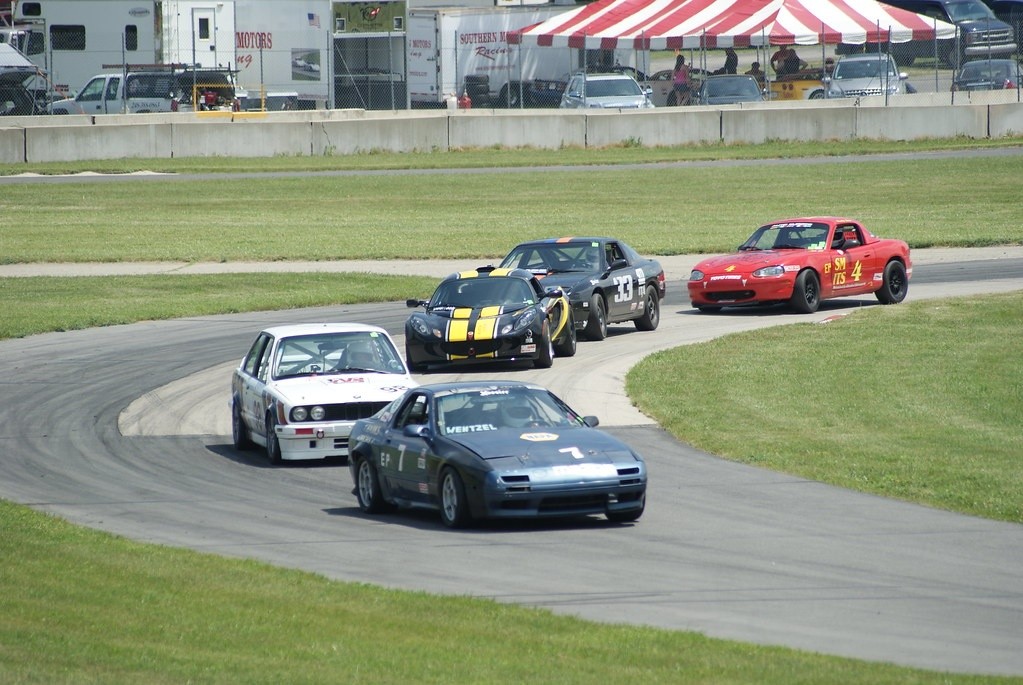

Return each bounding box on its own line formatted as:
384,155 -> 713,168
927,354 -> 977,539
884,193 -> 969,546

405,269 -> 579,373
228,320 -> 418,467
557,67 -> 706,111
700,73 -> 771,104
346,380 -> 648,534
499,235 -> 668,344
949,60 -> 1023,91
688,214 -> 912,316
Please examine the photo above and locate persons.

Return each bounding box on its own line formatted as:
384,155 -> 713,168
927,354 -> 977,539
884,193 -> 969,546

343,340 -> 379,370
744,61 -> 764,80
776,48 -> 808,73
722,47 -> 738,74
585,247 -> 611,270
671,54 -> 691,105
496,396 -> 539,428
821,227 -> 845,249
771,43 -> 790,80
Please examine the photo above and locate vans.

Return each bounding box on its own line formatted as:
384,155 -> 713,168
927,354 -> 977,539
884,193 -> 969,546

832,0 -> 1016,69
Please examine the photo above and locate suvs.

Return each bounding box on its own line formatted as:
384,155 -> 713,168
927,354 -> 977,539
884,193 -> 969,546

42,68 -> 242,114
818,53 -> 908,98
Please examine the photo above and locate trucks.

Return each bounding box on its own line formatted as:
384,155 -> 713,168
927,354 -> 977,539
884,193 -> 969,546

403,2 -> 645,109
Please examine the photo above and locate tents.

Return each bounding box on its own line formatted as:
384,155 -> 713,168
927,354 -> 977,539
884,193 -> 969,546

508,0 -> 960,111
